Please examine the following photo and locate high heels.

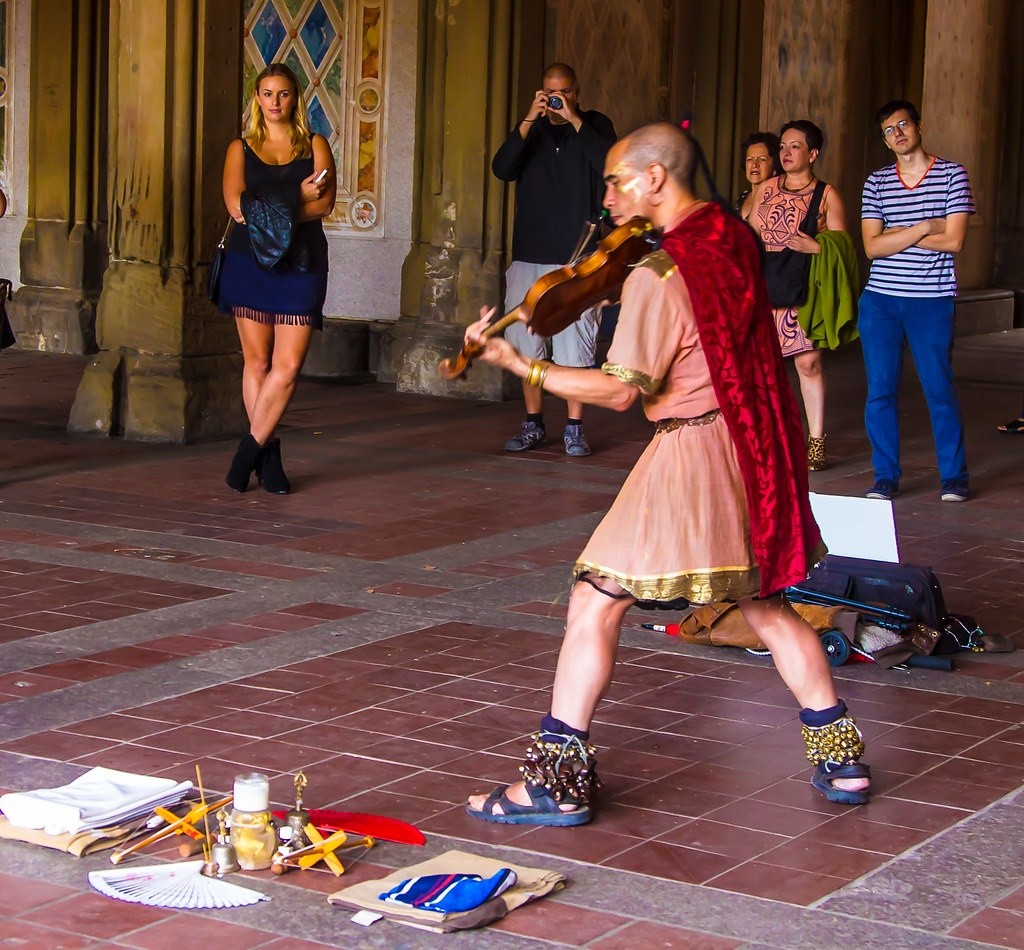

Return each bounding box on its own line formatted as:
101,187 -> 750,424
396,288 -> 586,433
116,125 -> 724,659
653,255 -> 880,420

807,433 -> 828,471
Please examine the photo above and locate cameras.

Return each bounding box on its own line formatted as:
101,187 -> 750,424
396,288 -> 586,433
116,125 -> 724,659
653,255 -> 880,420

540,95 -> 563,110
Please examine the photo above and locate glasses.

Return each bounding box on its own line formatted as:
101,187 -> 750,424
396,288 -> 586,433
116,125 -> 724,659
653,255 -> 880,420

881,119 -> 916,137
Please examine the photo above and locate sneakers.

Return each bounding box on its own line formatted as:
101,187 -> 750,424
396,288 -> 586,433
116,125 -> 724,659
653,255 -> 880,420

940,474 -> 968,502
563,425 -> 591,457
866,478 -> 901,499
505,421 -> 547,451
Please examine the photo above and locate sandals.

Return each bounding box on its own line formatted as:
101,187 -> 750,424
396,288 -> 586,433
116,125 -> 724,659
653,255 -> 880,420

811,760 -> 872,805
466,778 -> 591,826
997,416 -> 1024,434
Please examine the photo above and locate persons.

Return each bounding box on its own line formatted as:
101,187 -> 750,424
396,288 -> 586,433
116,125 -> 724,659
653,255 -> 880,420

733,131 -> 780,219
749,119 -> 846,470
857,99 -> 975,500
466,123 -> 871,827
219,63 -> 338,494
492,62 -> 619,458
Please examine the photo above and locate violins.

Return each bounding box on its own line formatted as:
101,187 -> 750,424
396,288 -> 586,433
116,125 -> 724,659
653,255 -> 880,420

437,215 -> 655,381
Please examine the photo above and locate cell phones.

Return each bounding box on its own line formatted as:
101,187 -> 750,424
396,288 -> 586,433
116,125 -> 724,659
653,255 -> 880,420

312,169 -> 328,183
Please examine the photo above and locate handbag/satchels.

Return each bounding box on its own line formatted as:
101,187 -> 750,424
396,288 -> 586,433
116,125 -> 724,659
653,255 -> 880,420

763,218 -> 817,307
0,277 -> 16,350
207,248 -> 224,307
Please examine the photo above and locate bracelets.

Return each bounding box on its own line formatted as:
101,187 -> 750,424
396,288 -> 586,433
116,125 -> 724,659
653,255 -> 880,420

524,120 -> 536,122
525,360 -> 549,389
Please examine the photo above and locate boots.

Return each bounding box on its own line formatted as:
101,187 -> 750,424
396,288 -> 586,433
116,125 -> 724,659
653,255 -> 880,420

255,438 -> 291,494
225,432 -> 261,495
